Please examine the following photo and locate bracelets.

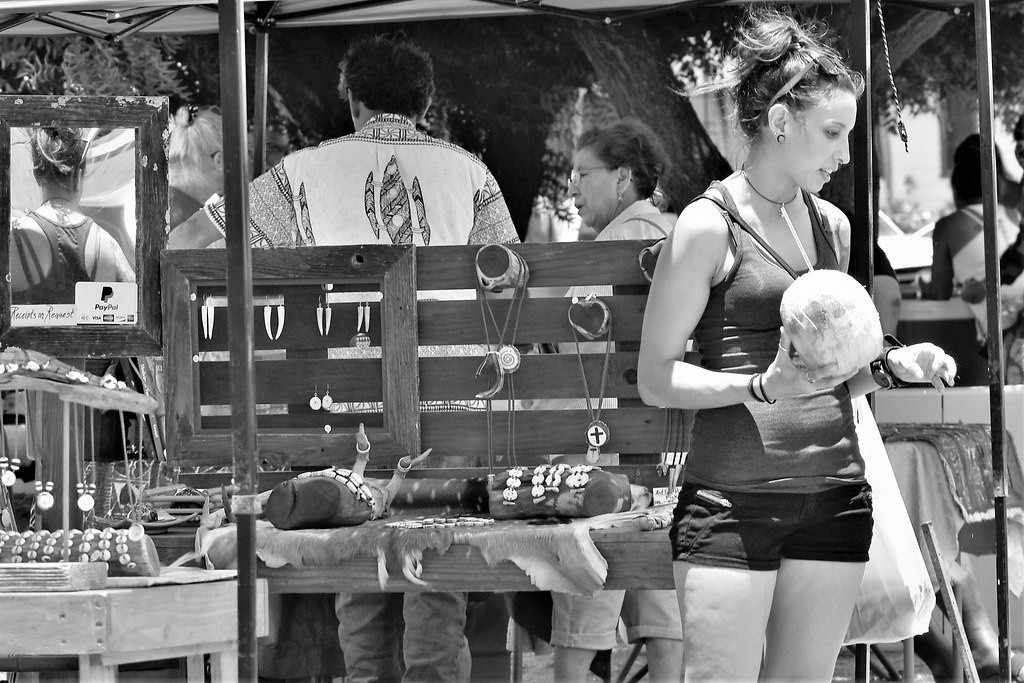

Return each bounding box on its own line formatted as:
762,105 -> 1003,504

747,372 -> 776,405
502,463 -> 606,509
0,528 -> 139,575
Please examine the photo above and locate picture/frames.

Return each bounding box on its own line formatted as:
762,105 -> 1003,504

157,245 -> 422,472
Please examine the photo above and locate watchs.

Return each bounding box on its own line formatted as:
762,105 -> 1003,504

870,334 -> 911,390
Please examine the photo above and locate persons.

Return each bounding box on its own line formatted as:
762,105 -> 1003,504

12,128 -> 137,307
636,15 -> 960,682
846,107 -> 1023,682
513,116 -> 701,682
174,31 -> 524,683
137,103 -> 222,456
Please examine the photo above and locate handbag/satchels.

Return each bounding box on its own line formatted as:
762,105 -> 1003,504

841,395 -> 936,647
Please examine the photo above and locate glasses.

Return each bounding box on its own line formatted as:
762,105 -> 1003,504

763,56 -> 855,127
568,166 -> 633,186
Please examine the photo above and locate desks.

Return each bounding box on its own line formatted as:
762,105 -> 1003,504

151,523 -> 677,683
0,577 -> 269,683
877,421 -> 1024,683
895,298 -> 990,389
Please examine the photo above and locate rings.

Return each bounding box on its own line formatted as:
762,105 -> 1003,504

778,343 -> 791,352
806,371 -> 818,384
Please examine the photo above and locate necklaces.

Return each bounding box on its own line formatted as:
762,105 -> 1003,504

475,252 -> 686,494
740,159 -> 798,218
0,361 -> 148,543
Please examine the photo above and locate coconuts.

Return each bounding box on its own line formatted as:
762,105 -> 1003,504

778,270 -> 883,371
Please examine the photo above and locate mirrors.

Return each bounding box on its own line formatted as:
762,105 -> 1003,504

0,95 -> 170,358
